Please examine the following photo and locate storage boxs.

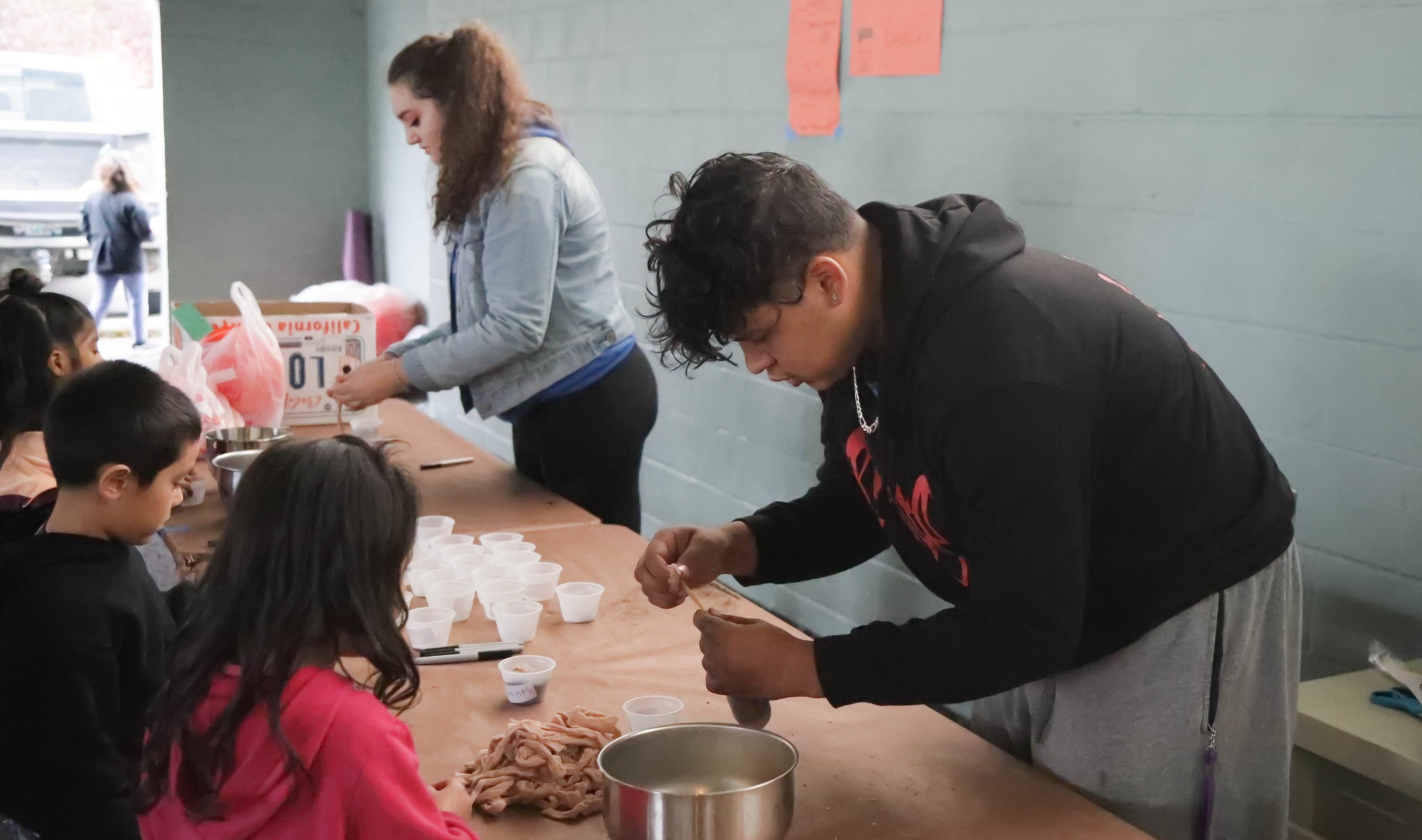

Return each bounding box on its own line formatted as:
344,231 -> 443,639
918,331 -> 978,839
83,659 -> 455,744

171,299 -> 377,426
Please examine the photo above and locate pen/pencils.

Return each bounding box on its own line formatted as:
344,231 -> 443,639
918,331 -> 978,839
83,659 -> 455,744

413,640 -> 523,665
420,456 -> 474,470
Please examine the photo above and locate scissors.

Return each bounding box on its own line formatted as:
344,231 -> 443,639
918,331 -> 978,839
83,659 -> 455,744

1370,685 -> 1422,721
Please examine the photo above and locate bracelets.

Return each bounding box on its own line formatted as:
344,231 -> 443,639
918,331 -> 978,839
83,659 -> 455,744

388,358 -> 411,395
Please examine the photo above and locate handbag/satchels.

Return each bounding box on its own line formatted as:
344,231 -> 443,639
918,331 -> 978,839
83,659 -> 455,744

202,280 -> 286,427
158,316 -> 244,454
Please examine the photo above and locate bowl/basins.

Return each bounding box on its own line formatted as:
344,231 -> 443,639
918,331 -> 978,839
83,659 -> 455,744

595,720 -> 800,840
203,425 -> 294,479
211,447 -> 271,509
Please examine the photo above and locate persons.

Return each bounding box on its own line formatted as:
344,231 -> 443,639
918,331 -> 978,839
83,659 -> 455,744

325,19 -> 660,537
634,151 -> 1307,840
0,269 -> 483,840
81,159 -> 155,348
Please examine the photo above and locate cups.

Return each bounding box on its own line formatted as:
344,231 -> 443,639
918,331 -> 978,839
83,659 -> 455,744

182,483 -> 206,506
395,515 -> 606,706
623,695 -> 684,732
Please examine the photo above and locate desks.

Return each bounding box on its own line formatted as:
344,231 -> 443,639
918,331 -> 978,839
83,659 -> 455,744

159,396 -> 1153,840
